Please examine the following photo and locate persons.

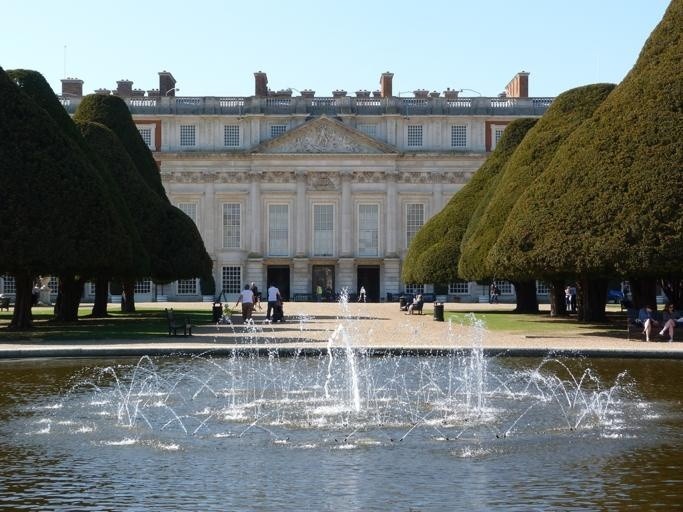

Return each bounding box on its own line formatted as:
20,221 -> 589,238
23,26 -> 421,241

637,304 -> 659,343
215,303 -> 232,326
569,285 -> 577,311
235,284 -> 256,325
400,289 -> 417,310
265,282 -> 282,319
659,300 -> 682,342
315,284 -> 323,302
489,283 -> 494,303
403,292 -> 422,316
493,286 -> 498,303
564,286 -> 572,311
249,282 -> 258,312
324,286 -> 334,301
31,281 -> 40,306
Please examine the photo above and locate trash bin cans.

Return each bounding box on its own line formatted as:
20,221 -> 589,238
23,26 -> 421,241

213,302 -> 223,322
400,296 -> 407,309
434,301 -> 444,321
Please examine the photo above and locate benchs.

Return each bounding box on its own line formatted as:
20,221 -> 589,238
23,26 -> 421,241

625,316 -> 680,341
165,307 -> 195,336
408,295 -> 424,315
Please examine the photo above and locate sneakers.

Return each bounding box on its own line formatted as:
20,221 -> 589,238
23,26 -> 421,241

660,330 -> 674,342
